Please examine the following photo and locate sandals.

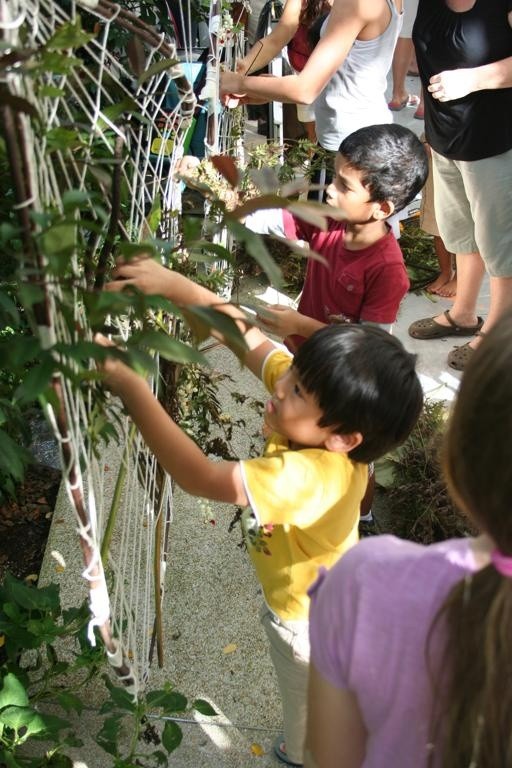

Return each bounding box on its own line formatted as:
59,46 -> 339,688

275,733 -> 299,766
387,94 -> 425,119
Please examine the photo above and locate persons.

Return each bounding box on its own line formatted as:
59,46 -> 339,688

167,121 -> 430,361
68,246 -> 423,767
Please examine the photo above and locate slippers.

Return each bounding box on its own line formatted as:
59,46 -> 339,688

447,331 -> 490,370
407,308 -> 486,340
